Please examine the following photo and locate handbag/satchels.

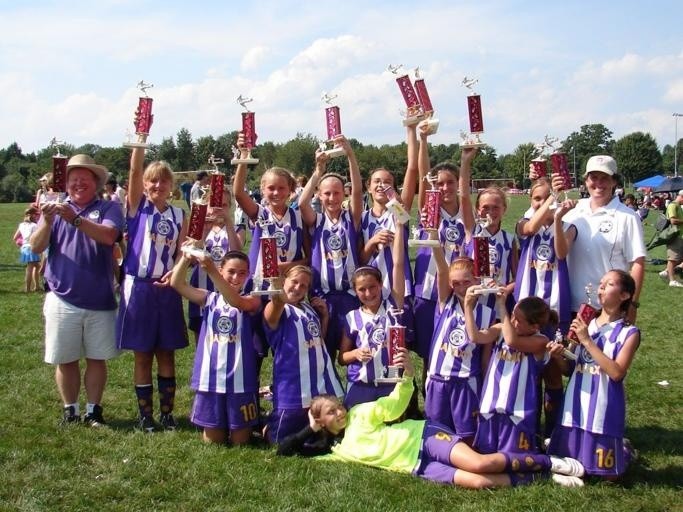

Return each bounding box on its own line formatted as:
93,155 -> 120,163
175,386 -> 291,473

656,214 -> 669,233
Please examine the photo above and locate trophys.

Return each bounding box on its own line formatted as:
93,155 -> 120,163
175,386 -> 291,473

412,64 -> 443,136
383,61 -> 426,126
121,77 -> 156,149
316,87 -> 348,157
458,75 -> 486,150
229,91 -> 262,168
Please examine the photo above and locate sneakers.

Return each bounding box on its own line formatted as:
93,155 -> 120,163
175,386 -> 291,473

622,436 -> 639,463
552,473 -> 584,489
548,453 -> 585,477
84,403 -> 110,429
62,404 -> 82,424
257,384 -> 274,401
138,414 -> 155,430
658,270 -> 668,278
160,411 -> 177,429
668,280 -> 683,288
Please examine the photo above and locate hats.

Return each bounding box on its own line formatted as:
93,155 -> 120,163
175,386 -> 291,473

585,154 -> 618,176
66,153 -> 109,188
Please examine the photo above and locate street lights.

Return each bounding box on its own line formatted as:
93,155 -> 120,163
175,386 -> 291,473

672,112 -> 683,178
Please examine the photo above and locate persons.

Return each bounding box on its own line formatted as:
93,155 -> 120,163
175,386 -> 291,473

632,174 -> 683,289
11,105 -> 648,491
